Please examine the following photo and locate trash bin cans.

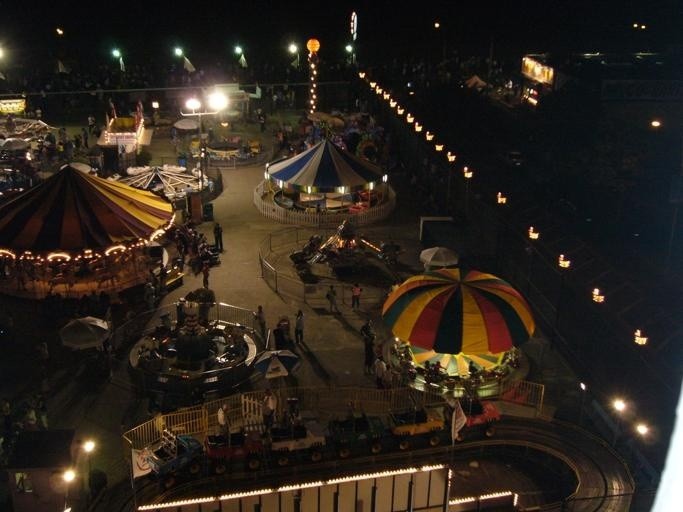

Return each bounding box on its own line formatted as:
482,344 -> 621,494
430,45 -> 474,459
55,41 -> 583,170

178,156 -> 186,166
204,203 -> 213,222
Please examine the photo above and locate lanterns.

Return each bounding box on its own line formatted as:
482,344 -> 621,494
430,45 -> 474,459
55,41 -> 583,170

529,226 -> 540,240
497,192 -> 507,204
307,38 -> 320,114
634,329 -> 649,345
592,288 -> 605,303
357,71 -> 474,178
559,254 -> 571,268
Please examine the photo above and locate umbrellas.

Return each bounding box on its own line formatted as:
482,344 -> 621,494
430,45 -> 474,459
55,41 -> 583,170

420,247 -> 458,266
61,316 -> 109,348
254,350 -> 299,387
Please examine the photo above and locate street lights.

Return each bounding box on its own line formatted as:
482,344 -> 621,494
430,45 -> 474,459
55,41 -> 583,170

181,92 -> 228,193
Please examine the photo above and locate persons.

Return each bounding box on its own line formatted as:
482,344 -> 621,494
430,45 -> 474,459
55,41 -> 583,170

258,114 -> 266,132
2,392 -> 48,430
257,306 -> 266,336
361,319 -> 477,388
217,404 -> 231,435
326,285 -> 337,312
351,283 -> 361,311
149,263 -> 168,294
175,222 -> 223,257
273,324 -> 283,350
271,122 -> 294,145
262,389 -> 277,430
0,107 -> 96,161
295,309 -> 303,344
203,261 -> 209,287
